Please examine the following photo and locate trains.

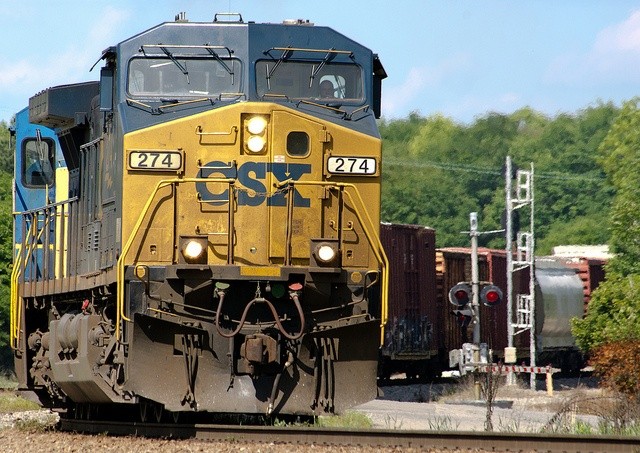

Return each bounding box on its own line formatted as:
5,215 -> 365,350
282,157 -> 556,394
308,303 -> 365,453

8,11 -> 610,424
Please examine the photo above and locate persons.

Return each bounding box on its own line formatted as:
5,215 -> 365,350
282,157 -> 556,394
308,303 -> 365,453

318,80 -> 339,98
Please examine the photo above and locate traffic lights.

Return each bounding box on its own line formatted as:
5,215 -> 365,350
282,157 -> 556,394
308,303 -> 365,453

450,305 -> 474,327
447,283 -> 471,305
480,284 -> 504,306
